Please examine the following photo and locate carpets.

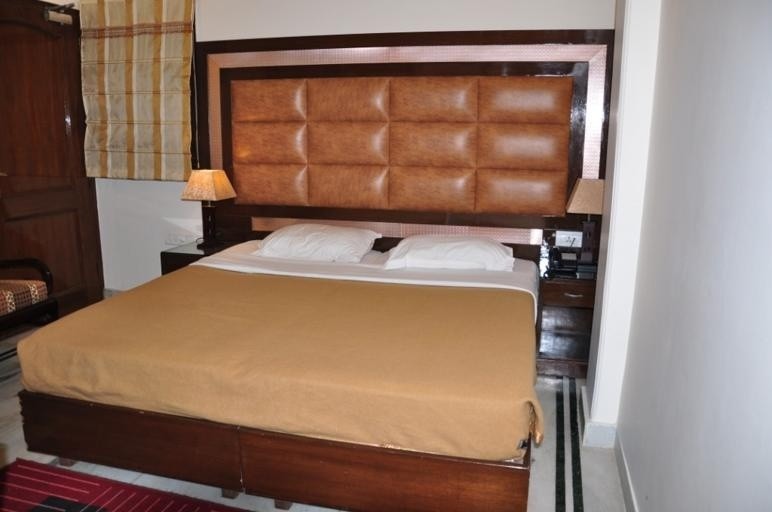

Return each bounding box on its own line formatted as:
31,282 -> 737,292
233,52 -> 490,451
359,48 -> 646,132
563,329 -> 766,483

1,456 -> 276,512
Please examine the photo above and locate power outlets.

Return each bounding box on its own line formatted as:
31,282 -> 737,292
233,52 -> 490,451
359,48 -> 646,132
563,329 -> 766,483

555,230 -> 583,248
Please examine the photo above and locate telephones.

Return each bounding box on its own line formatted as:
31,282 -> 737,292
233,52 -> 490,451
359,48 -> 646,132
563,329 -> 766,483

549,248 -> 578,272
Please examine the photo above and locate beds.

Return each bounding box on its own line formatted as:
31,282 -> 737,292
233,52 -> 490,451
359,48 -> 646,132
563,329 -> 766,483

16,61 -> 590,512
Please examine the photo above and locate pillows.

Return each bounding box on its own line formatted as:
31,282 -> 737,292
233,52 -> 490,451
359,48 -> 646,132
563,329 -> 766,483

250,223 -> 516,273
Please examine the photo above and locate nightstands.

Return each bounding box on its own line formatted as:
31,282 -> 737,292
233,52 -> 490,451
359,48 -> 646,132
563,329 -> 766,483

160,239 -> 226,276
535,277 -> 597,380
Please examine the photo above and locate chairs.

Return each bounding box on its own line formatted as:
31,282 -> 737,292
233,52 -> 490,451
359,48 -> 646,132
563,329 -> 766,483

0,255 -> 55,341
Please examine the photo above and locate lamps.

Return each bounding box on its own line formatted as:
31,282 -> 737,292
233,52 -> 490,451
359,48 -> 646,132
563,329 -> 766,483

180,168 -> 238,252
565,173 -> 606,259
